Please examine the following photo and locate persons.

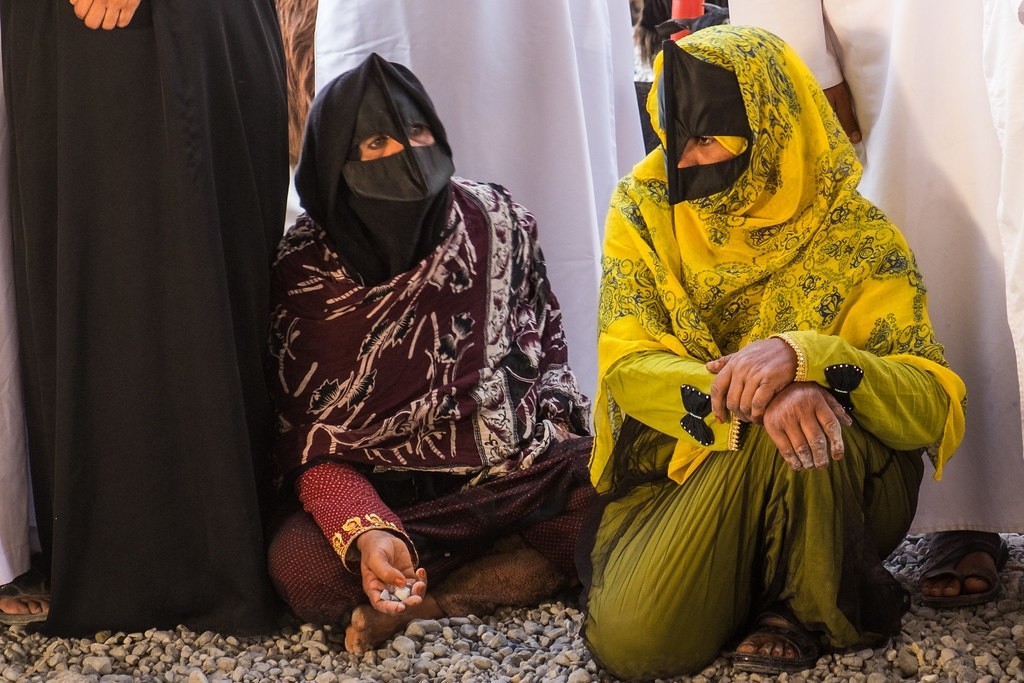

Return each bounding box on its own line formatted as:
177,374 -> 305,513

3,0 -> 650,635
264,55 -> 603,655
630,0 -> 732,155
729,1 -> 1024,605
590,28 -> 961,681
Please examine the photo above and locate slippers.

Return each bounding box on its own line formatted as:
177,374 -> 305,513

917,529 -> 1009,607
733,603 -> 819,672
0,580 -> 49,624
